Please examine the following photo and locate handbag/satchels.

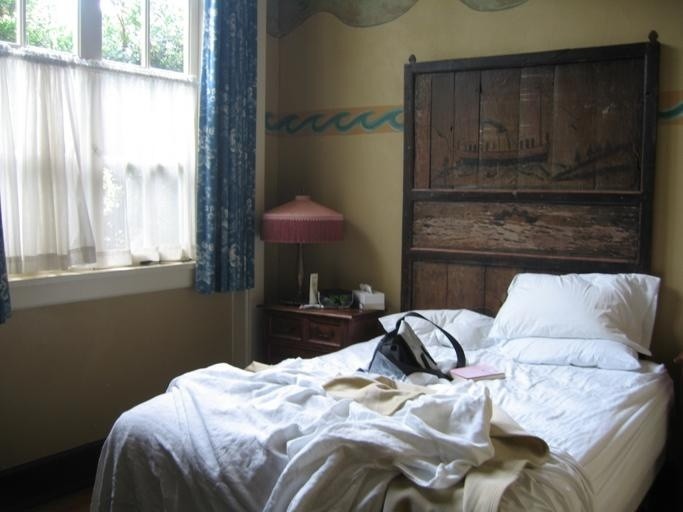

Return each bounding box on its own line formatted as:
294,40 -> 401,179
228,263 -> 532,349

356,312 -> 466,383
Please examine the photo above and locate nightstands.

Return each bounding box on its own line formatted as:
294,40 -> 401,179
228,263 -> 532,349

256,302 -> 384,365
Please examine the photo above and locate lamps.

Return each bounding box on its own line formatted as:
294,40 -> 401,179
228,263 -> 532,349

264,196 -> 344,307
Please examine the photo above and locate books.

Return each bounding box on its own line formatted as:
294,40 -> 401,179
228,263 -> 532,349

449,363 -> 507,382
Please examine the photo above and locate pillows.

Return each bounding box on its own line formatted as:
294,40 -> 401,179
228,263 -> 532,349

487,273 -> 661,355
377,309 -> 494,350
496,339 -> 643,370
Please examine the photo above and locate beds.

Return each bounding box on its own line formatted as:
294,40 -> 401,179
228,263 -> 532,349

93,312 -> 673,512
